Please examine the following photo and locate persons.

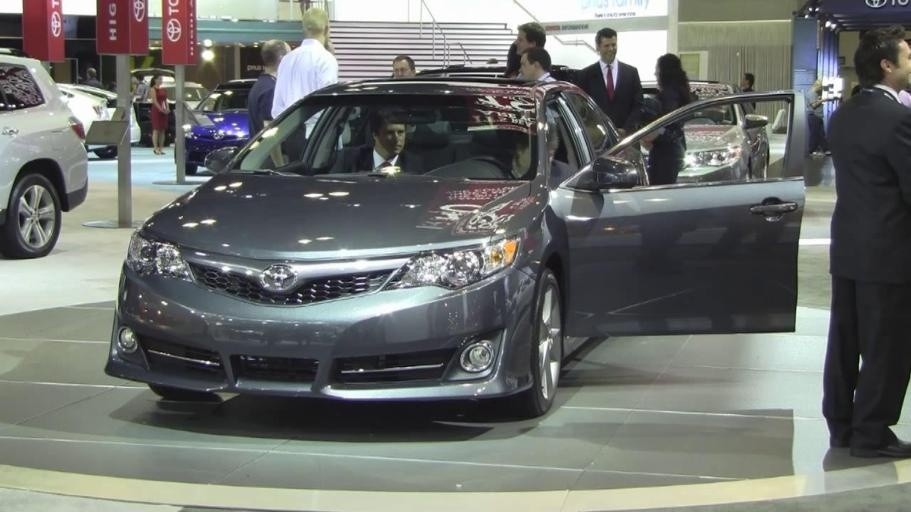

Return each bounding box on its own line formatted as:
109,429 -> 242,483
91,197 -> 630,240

82,67 -> 104,88
740,73 -> 755,115
515,22 -> 547,56
575,28 -> 645,151
518,48 -> 557,82
647,53 -> 691,186
247,40 -> 291,138
335,109 -> 417,175
132,74 -> 148,145
805,80 -> 832,156
496,127 -> 570,188
150,74 -> 170,155
271,8 -> 338,119
393,56 -> 416,77
505,42 -> 522,76
822,26 -> 911,459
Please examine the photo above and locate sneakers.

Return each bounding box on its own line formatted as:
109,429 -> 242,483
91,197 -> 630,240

808,149 -> 833,158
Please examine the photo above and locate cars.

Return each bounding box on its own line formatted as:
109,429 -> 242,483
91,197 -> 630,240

175,76 -> 260,178
641,80 -> 770,186
107,81 -> 811,420
56,80 -> 143,159
131,67 -> 212,148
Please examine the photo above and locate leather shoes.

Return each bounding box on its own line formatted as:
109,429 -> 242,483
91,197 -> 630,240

153,149 -> 166,155
829,426 -> 851,448
849,425 -> 911,458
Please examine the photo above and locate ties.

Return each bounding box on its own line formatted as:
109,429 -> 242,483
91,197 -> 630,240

606,64 -> 615,102
381,161 -> 391,170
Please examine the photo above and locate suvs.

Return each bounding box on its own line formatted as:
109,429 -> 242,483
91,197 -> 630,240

416,65 -> 573,82
0,47 -> 92,257
